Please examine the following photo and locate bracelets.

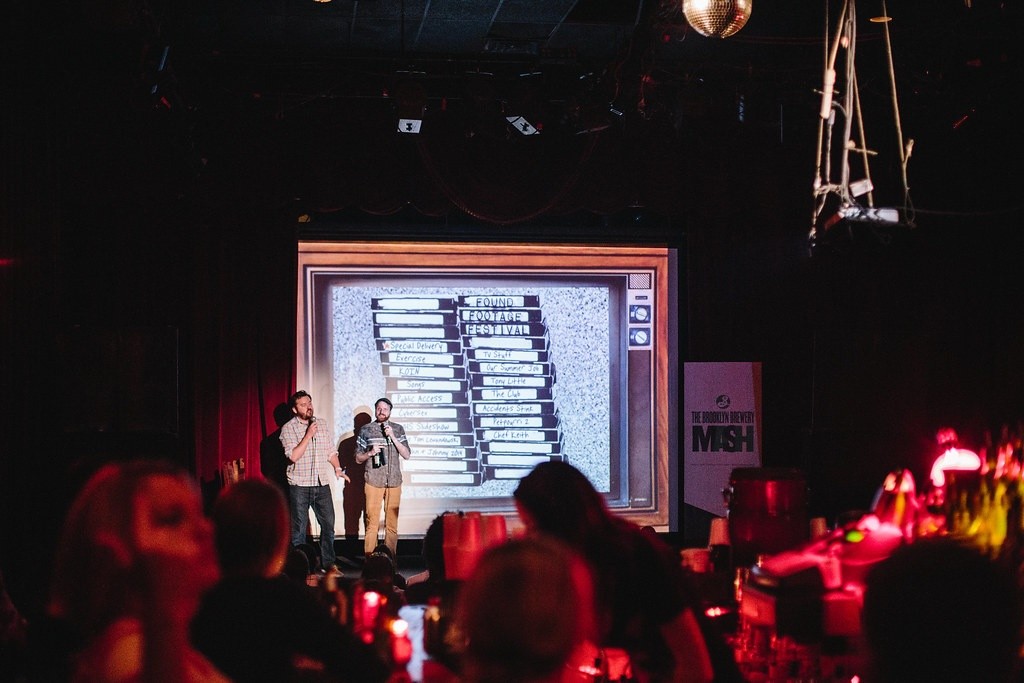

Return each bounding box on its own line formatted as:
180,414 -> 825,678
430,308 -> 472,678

336,467 -> 342,471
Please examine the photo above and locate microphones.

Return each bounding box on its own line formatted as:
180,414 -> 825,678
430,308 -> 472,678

384,422 -> 392,444
311,416 -> 316,441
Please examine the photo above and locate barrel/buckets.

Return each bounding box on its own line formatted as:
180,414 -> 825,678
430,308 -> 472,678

720,462 -> 813,561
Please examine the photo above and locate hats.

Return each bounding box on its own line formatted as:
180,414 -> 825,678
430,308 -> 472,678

368,545 -> 392,566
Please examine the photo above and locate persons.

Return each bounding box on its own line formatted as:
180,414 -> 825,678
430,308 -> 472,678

460,462 -> 1024,683
353,397 -> 411,566
279,390 -> 351,576
0,456 -> 461,683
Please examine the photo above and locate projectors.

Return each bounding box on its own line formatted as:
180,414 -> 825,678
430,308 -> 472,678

825,207 -> 899,230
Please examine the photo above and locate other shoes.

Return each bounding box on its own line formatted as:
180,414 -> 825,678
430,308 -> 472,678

321,565 -> 345,578
